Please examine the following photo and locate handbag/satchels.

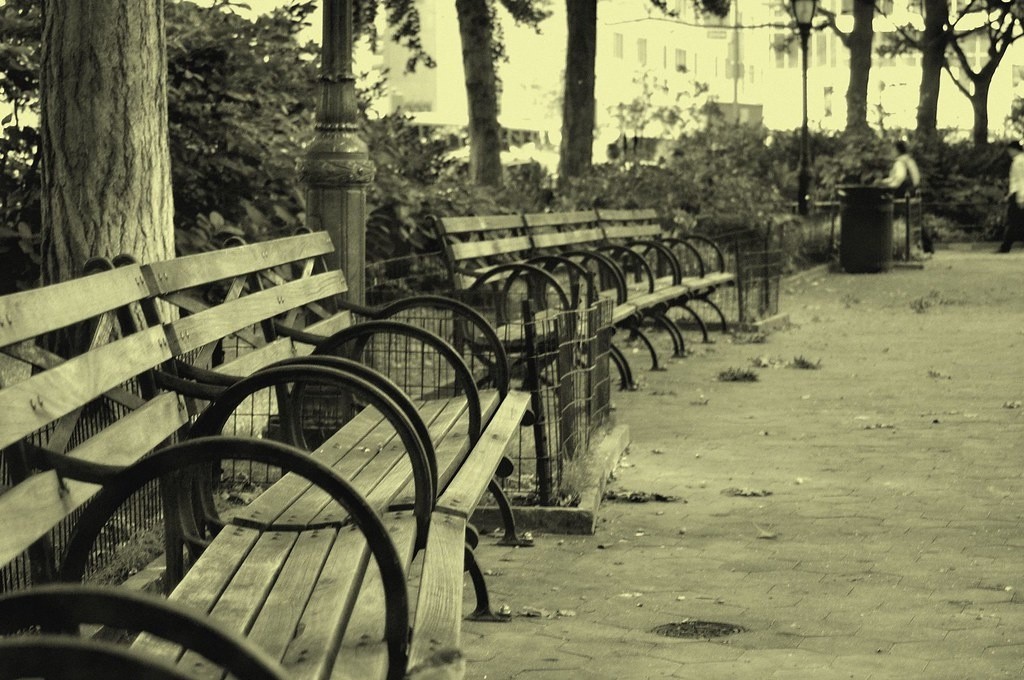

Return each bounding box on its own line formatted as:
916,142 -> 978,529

892,160 -> 915,200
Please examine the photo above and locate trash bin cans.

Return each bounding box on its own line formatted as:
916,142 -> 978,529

834,184 -> 896,274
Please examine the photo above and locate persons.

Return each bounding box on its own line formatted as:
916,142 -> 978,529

883,140 -> 934,254
996,140 -> 1024,253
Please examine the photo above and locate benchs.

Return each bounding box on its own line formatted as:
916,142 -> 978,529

426,207 -> 737,413
0,228 -> 537,680
